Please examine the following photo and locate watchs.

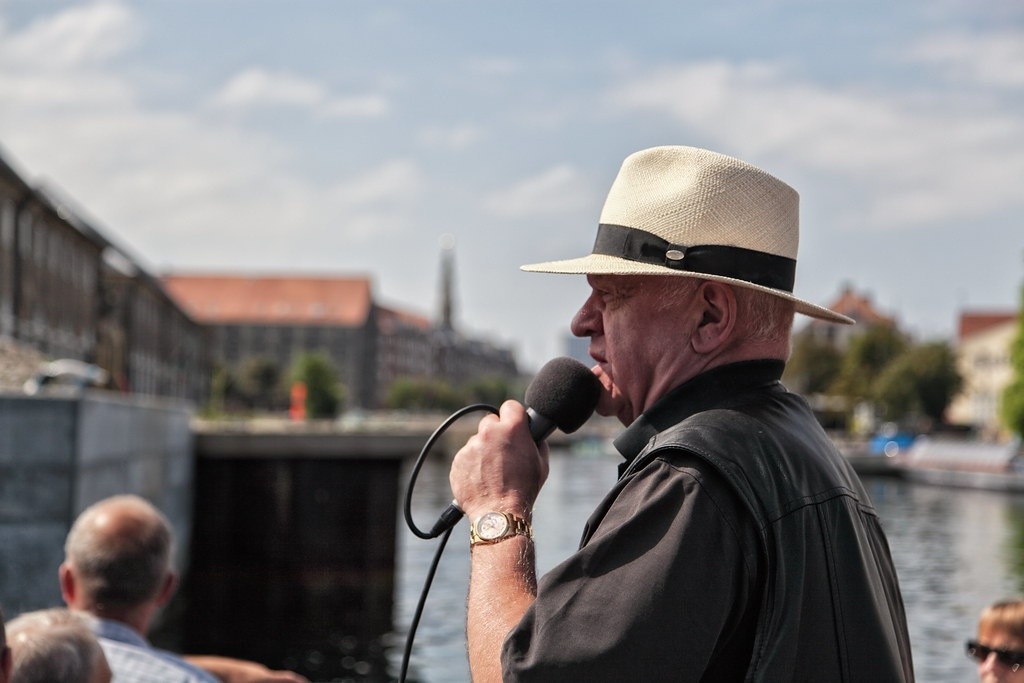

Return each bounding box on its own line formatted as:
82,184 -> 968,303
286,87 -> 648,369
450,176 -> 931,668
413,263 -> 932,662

469,511 -> 533,553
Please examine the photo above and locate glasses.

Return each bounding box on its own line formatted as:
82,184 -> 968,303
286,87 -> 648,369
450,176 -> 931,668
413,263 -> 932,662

965,637 -> 1024,667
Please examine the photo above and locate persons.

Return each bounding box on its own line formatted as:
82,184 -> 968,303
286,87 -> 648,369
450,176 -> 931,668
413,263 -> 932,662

0,491 -> 224,683
965,599 -> 1024,683
447,145 -> 915,683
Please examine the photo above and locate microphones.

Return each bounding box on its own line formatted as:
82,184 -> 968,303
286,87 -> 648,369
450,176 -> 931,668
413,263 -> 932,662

429,356 -> 603,539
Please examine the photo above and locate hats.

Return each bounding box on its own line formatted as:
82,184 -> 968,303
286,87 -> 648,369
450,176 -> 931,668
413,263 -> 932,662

520,145 -> 858,325
